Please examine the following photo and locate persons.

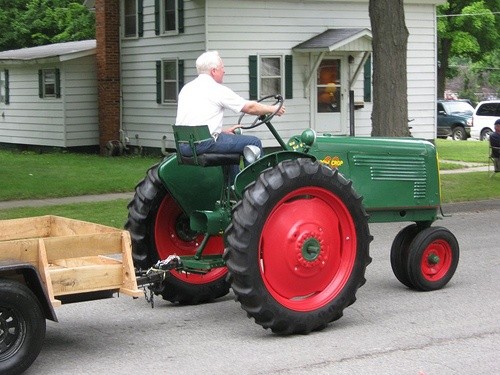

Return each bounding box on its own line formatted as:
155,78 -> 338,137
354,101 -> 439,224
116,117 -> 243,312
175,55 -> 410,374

174,50 -> 285,191
489,118 -> 500,172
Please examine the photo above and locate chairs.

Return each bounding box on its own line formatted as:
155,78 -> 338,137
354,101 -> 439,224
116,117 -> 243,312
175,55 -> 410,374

172,124 -> 241,209
488,146 -> 500,181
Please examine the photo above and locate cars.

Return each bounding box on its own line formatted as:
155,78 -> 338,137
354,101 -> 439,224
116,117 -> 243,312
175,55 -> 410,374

471,100 -> 500,141
437,99 -> 475,140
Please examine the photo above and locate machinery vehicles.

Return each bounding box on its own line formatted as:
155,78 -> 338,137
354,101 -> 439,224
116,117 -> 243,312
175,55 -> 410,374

1,94 -> 459,375
